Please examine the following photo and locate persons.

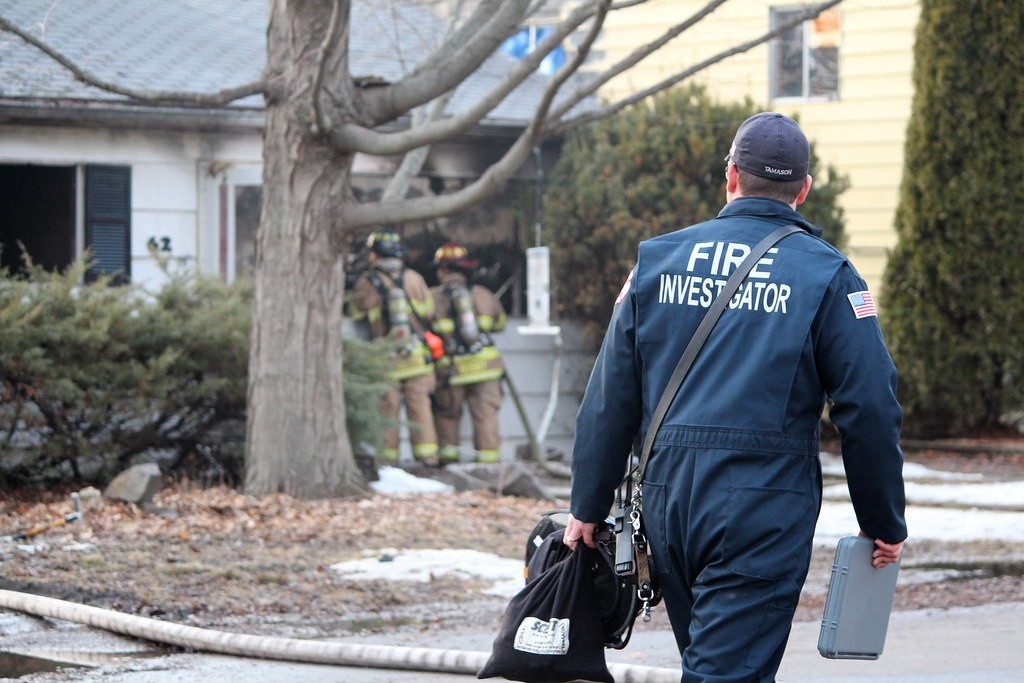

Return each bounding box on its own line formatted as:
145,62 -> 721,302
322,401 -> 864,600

428,242 -> 507,468
331,225 -> 439,469
563,112 -> 908,683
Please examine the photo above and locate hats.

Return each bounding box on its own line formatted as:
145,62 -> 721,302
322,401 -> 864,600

725,111 -> 811,183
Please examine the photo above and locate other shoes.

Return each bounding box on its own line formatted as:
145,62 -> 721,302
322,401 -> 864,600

438,454 -> 461,467
422,454 -> 440,467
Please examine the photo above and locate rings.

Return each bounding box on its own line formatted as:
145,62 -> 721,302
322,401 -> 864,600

568,537 -> 580,542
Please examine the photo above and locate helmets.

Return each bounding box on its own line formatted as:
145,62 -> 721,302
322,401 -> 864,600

362,225 -> 405,259
525,508 -> 646,650
430,240 -> 480,271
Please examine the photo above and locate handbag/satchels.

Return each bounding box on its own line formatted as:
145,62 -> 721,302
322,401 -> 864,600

609,472 -> 664,608
